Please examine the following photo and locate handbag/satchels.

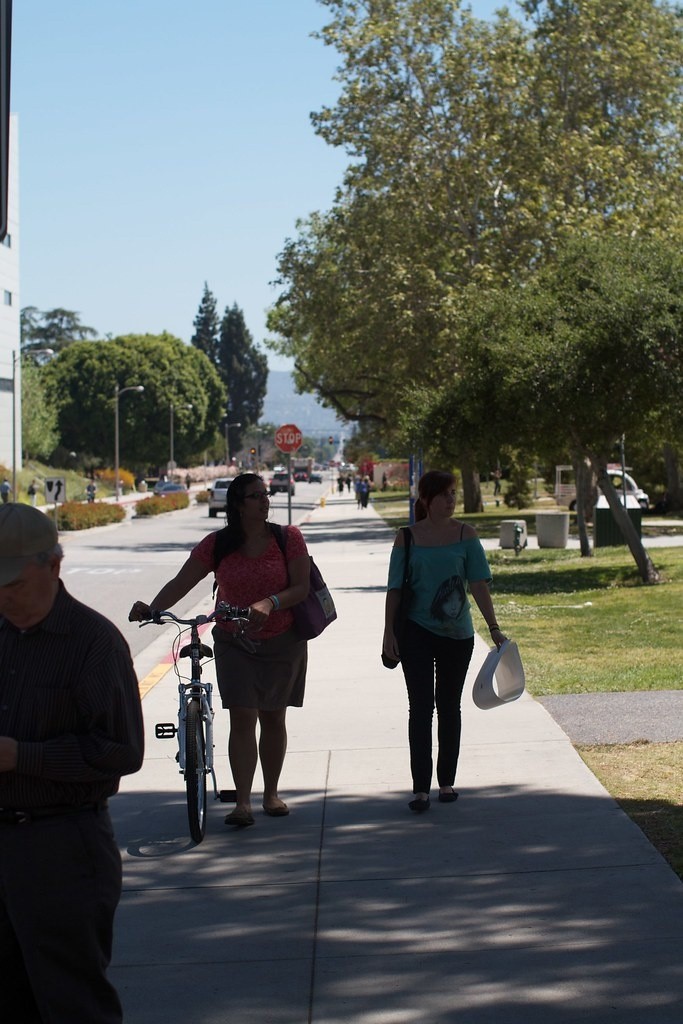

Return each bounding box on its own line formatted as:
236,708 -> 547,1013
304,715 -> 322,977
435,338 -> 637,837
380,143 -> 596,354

381,527 -> 411,670
279,526 -> 337,639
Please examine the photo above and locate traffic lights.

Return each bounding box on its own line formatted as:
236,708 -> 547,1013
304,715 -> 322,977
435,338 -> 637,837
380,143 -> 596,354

251,448 -> 255,455
329,437 -> 333,445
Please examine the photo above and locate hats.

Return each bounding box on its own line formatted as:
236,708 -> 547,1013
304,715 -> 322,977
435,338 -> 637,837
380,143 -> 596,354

0,503 -> 59,559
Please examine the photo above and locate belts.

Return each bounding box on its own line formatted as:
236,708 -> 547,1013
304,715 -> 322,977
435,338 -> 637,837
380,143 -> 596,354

1,801 -> 101,826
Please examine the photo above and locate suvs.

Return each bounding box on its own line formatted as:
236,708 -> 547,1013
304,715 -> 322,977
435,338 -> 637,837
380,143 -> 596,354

207,478 -> 235,518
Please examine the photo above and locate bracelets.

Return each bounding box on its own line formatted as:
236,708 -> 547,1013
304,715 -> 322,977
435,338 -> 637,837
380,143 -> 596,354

490,628 -> 500,632
266,595 -> 279,611
489,623 -> 499,627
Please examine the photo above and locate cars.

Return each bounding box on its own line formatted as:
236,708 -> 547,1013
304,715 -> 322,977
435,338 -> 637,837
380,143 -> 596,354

555,464 -> 650,512
294,459 -> 341,484
153,480 -> 189,497
269,471 -> 295,496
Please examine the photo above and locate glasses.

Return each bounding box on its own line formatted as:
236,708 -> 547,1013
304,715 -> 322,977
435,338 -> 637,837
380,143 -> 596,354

244,491 -> 273,499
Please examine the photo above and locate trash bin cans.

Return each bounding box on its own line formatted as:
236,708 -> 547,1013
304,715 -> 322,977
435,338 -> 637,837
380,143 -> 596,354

595,508 -> 640,548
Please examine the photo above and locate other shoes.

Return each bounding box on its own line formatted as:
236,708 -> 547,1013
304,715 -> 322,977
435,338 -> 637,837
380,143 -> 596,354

261,798 -> 289,815
438,787 -> 458,804
225,808 -> 255,824
409,795 -> 432,811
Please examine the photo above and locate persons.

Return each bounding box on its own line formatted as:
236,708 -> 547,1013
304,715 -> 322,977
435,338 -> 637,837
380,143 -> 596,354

0,477 -> 12,503
338,475 -> 351,491
382,473 -> 387,487
87,480 -> 95,504
186,473 -> 191,489
29,480 -> 37,506
0,503 -> 144,1024
128,474 -> 308,828
355,479 -> 370,510
383,470 -> 509,811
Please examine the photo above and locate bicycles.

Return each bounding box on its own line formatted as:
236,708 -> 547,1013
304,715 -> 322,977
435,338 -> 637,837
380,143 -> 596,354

136,605 -> 253,842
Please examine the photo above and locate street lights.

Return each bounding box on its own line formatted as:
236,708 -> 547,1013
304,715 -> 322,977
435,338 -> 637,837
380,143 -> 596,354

114,386 -> 145,502
11,348 -> 54,502
225,423 -> 241,464
169,401 -> 193,482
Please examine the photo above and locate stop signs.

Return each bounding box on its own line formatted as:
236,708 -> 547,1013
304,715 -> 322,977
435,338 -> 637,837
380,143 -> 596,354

275,424 -> 303,453
